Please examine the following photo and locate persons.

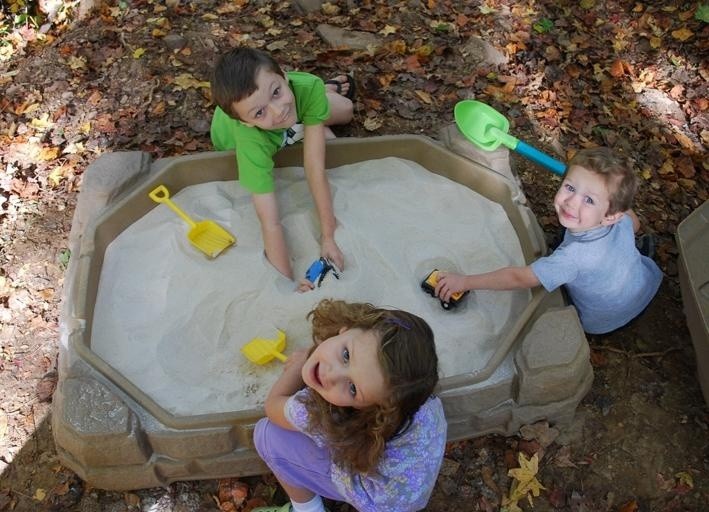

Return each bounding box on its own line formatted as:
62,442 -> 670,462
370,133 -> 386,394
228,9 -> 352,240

211,44 -> 356,294
435,147 -> 665,337
253,298 -> 448,512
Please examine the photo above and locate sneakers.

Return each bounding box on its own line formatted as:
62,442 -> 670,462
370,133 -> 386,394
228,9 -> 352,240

251,502 -> 330,512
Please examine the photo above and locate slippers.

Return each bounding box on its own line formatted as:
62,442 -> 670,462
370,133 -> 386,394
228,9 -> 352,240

324,74 -> 354,98
